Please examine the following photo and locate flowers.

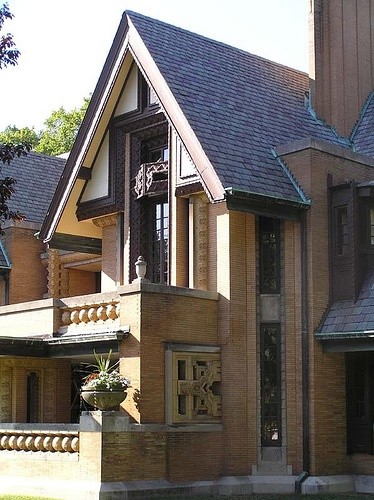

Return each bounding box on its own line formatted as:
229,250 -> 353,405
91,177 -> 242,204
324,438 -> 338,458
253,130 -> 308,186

76,348 -> 133,391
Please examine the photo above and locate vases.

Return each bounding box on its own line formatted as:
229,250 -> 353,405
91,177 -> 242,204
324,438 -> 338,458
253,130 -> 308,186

80,386 -> 127,411
